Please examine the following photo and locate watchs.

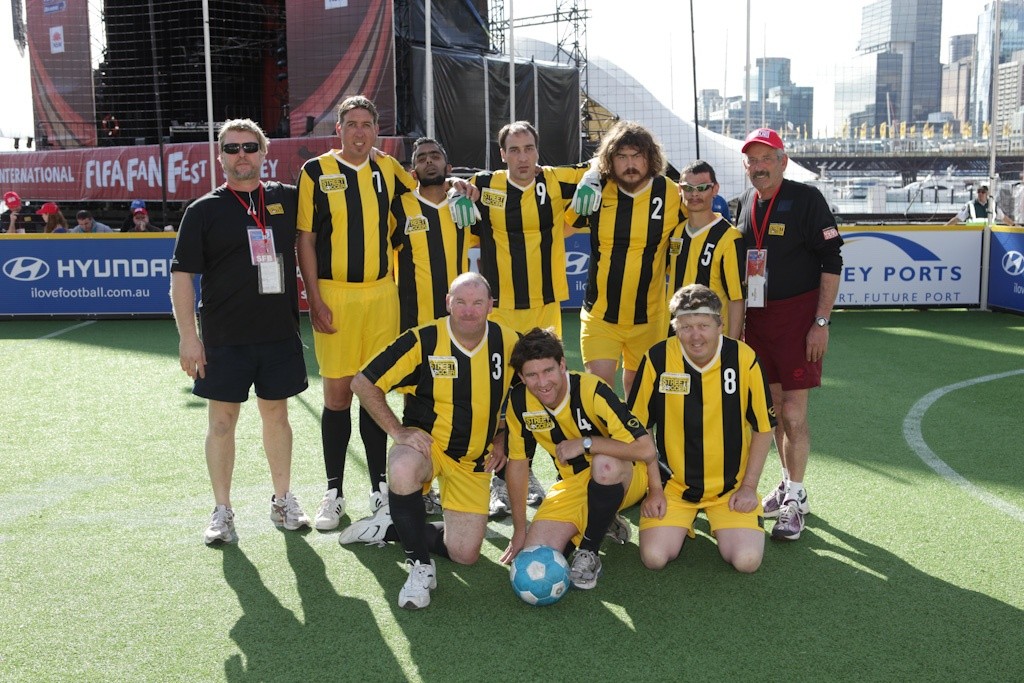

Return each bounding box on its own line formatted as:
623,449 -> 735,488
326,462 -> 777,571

814,316 -> 832,328
584,435 -> 592,455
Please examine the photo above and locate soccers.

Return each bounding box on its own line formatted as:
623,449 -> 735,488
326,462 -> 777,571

509,545 -> 570,607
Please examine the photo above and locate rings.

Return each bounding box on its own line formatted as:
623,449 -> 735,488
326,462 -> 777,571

489,454 -> 494,458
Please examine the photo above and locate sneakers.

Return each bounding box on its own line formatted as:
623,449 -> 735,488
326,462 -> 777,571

489,476 -> 512,517
314,488 -> 347,530
604,514 -> 632,545
569,550 -> 603,589
772,491 -> 807,541
270,491 -> 311,531
339,503 -> 395,548
761,481 -> 789,517
422,489 -> 442,515
397,558 -> 438,610
205,504 -> 236,544
526,467 -> 546,506
368,482 -> 389,512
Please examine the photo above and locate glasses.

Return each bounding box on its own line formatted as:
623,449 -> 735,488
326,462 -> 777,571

679,180 -> 714,192
223,142 -> 260,155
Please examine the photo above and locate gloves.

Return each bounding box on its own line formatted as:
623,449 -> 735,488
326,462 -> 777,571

447,187 -> 482,229
570,170 -> 603,217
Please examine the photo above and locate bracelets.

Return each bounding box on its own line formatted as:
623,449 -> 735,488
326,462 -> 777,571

1012,223 -> 1015,227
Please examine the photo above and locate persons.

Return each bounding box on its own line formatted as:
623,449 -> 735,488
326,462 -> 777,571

628,284 -> 779,572
499,328 -> 657,591
350,272 -> 525,609
35,202 -> 69,232
663,159 -> 748,342
168,119 -> 310,547
734,128 -> 845,540
562,123 -> 690,398
70,210 -> 113,233
945,186 -> 1022,226
0,192 -> 47,233
120,198 -> 162,232
388,121 -> 605,518
298,98 -> 481,531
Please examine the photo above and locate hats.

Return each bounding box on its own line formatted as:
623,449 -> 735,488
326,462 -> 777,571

978,185 -> 988,191
3,192 -> 20,209
130,200 -> 145,209
741,128 -> 784,154
133,209 -> 148,216
35,202 -> 59,216
